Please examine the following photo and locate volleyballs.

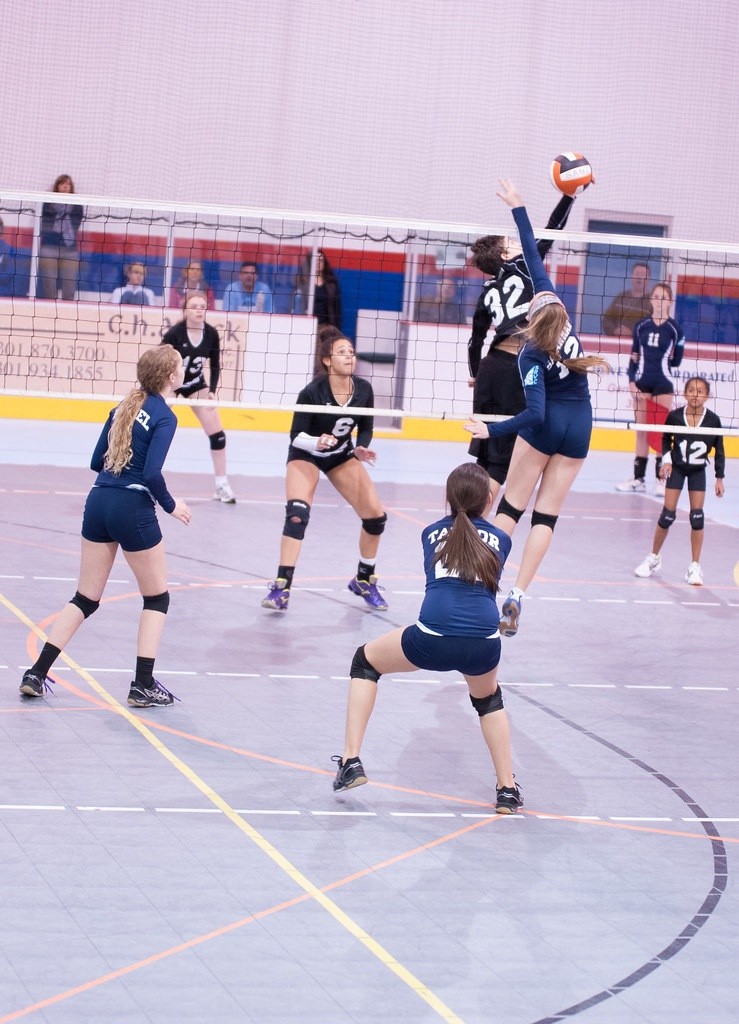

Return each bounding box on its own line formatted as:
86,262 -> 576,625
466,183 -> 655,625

549,150 -> 593,196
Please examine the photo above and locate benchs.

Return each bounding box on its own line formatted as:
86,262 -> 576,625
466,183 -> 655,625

57,287 -> 225,311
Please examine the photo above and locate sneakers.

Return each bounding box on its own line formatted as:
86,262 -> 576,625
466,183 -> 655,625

495,774 -> 524,814
616,478 -> 646,492
499,591 -> 522,638
347,575 -> 389,611
635,553 -> 661,576
214,485 -> 236,503
331,755 -> 367,793
20,669 -> 56,696
655,478 -> 666,496
685,561 -> 703,585
261,578 -> 290,612
128,678 -> 180,707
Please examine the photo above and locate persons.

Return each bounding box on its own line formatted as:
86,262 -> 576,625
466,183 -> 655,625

289,249 -> 343,380
19,344 -> 192,707
634,377 -> 725,585
602,262 -> 653,336
414,274 -> 466,324
261,324 -> 390,611
109,262 -> 155,305
222,261 -> 275,314
614,282 -> 684,497
330,462 -> 524,813
466,175 -> 596,522
0,217 -> 13,296
159,290 -> 237,503
170,259 -> 215,310
40,174 -> 83,300
461,178 -> 593,638
